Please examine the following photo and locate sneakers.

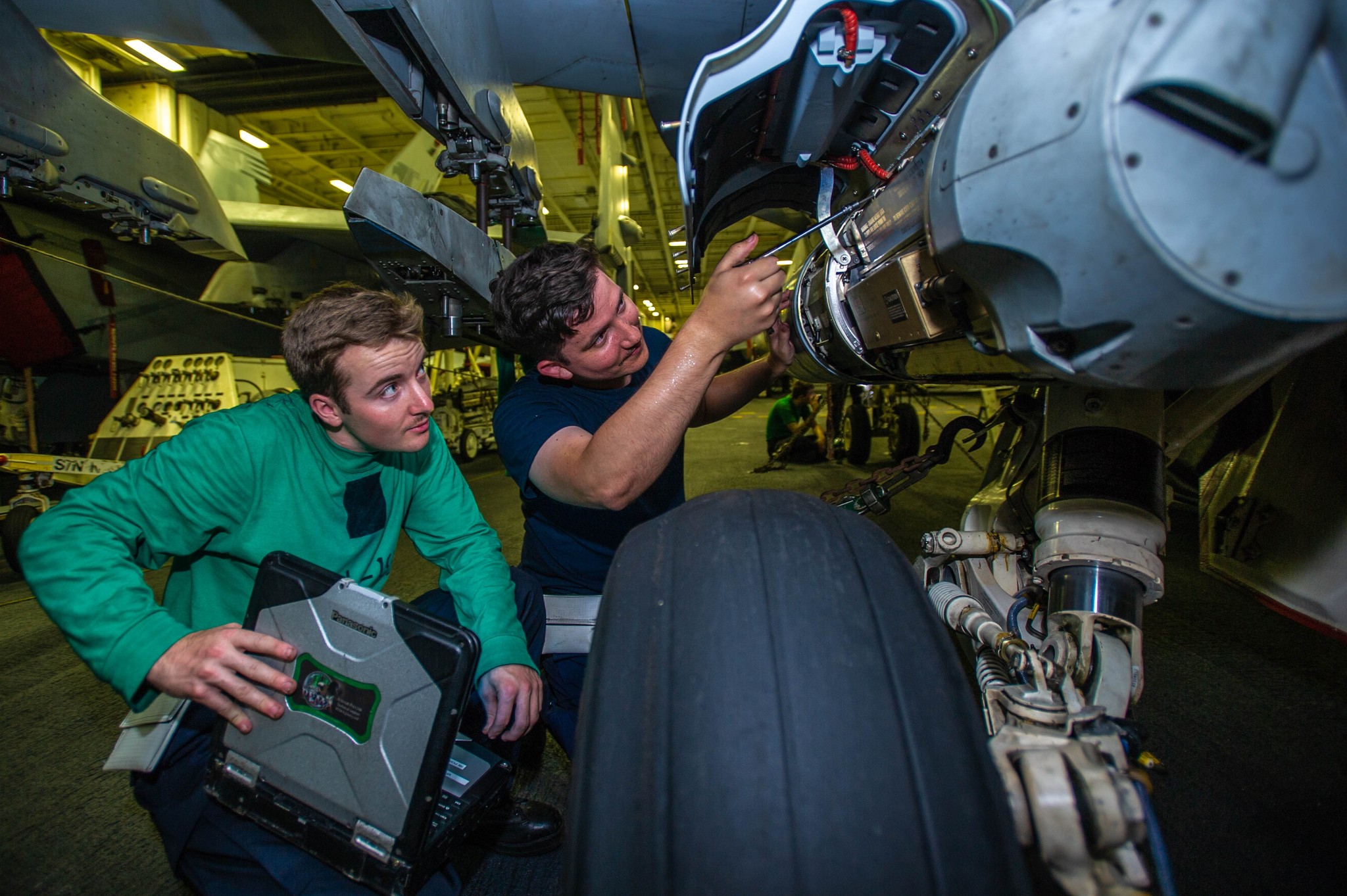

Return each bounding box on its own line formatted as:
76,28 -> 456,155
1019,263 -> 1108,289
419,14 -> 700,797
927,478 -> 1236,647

469,795 -> 565,856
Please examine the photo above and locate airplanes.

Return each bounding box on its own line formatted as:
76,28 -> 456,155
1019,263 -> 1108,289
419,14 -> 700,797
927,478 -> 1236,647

0,0 -> 1347,896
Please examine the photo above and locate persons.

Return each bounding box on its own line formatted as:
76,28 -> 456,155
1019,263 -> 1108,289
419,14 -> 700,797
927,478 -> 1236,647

766,380 -> 828,464
16,282 -> 562,896
489,232 -> 794,764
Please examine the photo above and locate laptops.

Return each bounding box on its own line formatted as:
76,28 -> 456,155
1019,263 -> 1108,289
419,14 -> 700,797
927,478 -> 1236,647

211,548 -> 514,864
